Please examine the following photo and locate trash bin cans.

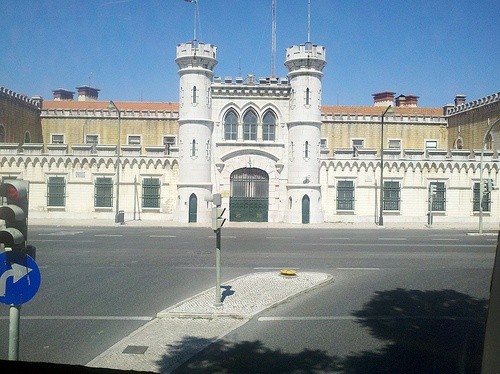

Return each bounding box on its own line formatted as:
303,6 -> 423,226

118,210 -> 126,224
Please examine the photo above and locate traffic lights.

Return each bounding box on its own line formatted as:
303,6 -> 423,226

1,182 -> 29,249
212,207 -> 226,230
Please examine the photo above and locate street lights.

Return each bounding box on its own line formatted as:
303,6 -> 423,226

378,105 -> 393,224
107,100 -> 122,223
